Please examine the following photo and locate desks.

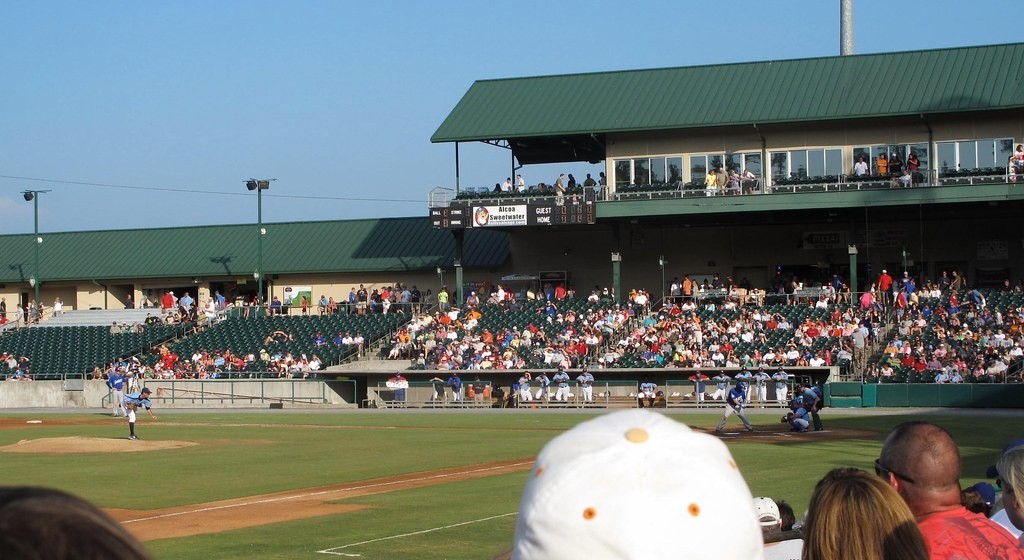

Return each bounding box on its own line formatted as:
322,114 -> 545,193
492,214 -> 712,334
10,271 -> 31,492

740,178 -> 763,195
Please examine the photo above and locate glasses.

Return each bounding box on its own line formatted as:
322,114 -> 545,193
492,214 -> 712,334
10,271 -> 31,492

873,458 -> 915,483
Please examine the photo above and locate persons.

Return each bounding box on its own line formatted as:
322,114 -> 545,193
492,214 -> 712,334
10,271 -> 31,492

14,304 -> 24,332
109,367 -> 128,417
0,486 -> 152,560
93,269 -> 1024,433
704,167 -> 755,196
802,467 -> 929,560
26,299 -> 44,325
1009,145 -> 1024,181
54,297 -> 63,317
879,423 -> 1024,560
528,172 -> 606,204
754,496 -> 804,560
126,294 -> 135,309
512,409 -> 763,560
494,174 -> 525,194
121,387 -> 157,440
852,153 -> 918,187
0,352 -> 32,381
0,297 -> 8,331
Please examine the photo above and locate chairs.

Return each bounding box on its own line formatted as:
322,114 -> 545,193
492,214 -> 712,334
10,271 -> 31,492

0,290 -> 1024,384
615,182 -> 740,197
771,172 -> 925,193
449,183 -> 583,207
939,160 -> 1024,185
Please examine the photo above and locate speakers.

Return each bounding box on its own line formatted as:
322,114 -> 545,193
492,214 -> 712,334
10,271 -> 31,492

24,193 -> 34,201
247,182 -> 257,190
260,182 -> 270,189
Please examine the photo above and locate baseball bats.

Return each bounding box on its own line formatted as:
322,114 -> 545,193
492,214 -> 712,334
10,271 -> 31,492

743,381 -> 751,412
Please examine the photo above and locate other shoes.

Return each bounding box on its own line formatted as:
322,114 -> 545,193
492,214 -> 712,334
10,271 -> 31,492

114,413 -> 119,417
816,427 -> 823,431
129,435 -> 139,441
749,429 -> 753,432
716,429 -> 720,432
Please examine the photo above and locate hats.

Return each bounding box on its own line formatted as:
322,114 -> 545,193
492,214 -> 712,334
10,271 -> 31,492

736,381 -> 744,389
512,407 -> 766,560
758,367 -> 763,370
115,368 -> 120,371
973,482 -> 995,506
721,370 -> 725,373
696,370 -> 701,374
142,388 -> 152,393
742,366 -> 747,370
778,367 -> 783,371
583,368 -> 587,372
986,439 -> 1024,478
753,496 -> 782,526
796,383 -> 803,390
559,368 -> 563,370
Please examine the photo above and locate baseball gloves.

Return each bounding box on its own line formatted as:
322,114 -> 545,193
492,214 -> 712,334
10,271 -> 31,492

781,415 -> 788,423
125,402 -> 134,410
